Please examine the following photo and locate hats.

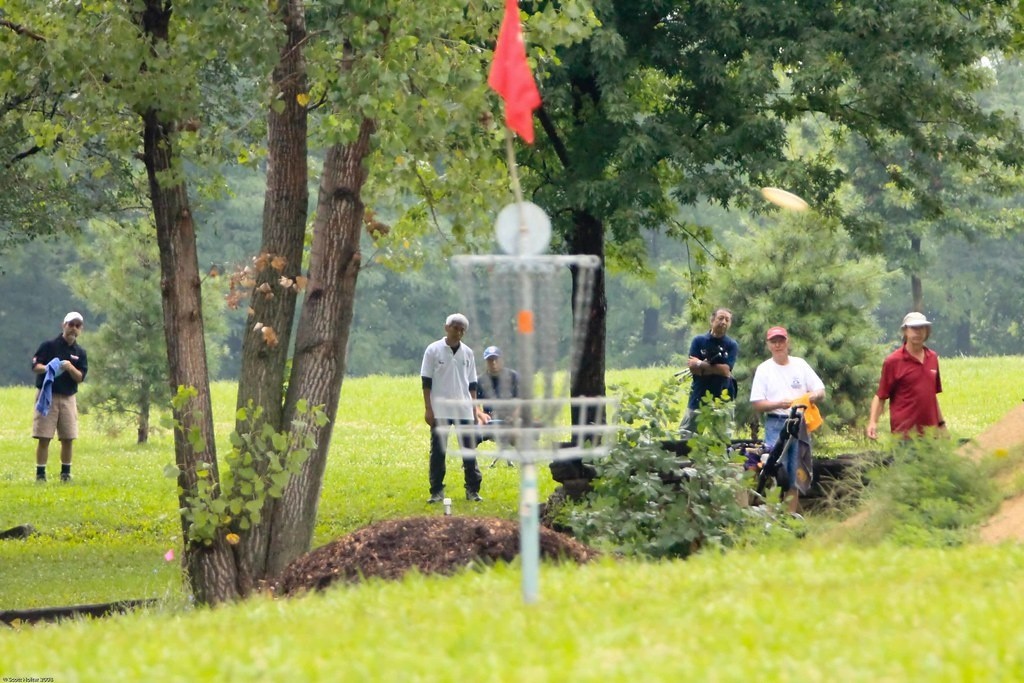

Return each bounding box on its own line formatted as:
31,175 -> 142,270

902,312 -> 931,328
65,312 -> 83,324
766,326 -> 788,341
483,346 -> 501,360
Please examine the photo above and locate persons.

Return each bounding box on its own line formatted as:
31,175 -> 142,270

867,312 -> 950,447
460,345 -> 521,471
671,307 -> 738,493
31,312 -> 88,484
750,326 -> 826,515
420,313 -> 484,504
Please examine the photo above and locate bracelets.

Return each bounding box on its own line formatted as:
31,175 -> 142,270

696,360 -> 702,369
938,420 -> 945,427
815,391 -> 819,400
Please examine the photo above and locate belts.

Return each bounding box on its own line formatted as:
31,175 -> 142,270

766,413 -> 789,418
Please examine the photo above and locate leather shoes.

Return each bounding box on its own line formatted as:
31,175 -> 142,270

428,492 -> 444,502
465,489 -> 483,502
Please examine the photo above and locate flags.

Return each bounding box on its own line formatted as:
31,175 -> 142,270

487,0 -> 542,144
